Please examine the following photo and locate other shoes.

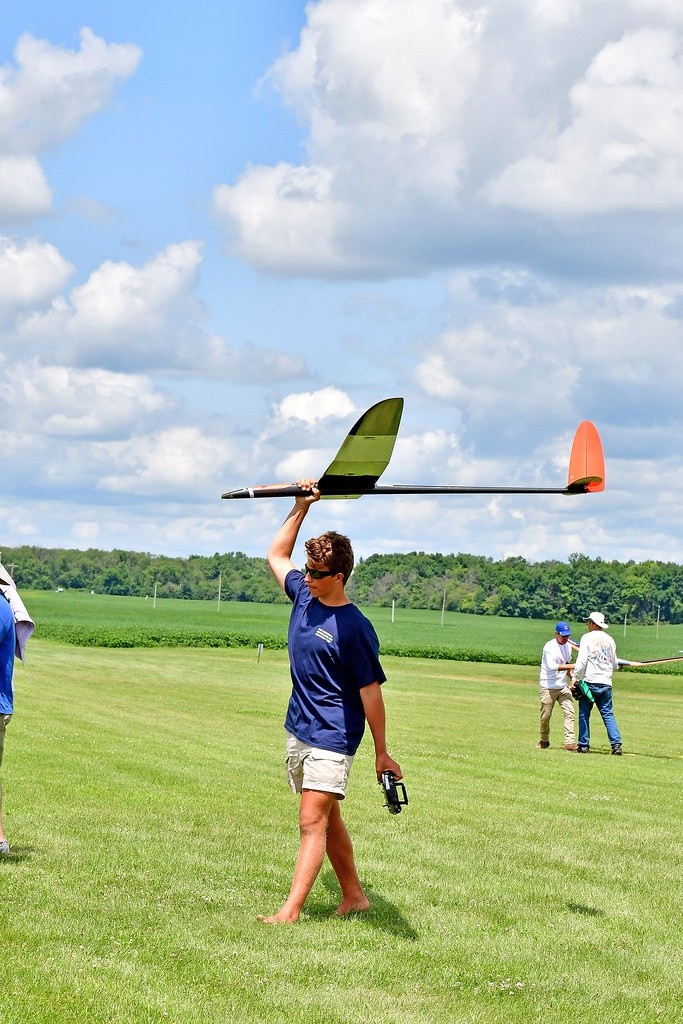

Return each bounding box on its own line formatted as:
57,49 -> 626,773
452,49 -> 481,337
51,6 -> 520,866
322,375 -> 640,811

540,741 -> 549,748
0,841 -> 9,854
578,745 -> 589,753
566,743 -> 578,751
611,747 -> 622,755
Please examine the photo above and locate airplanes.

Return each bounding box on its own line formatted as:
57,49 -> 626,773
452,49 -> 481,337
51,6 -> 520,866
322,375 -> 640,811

568,639 -> 683,666
221,398 -> 606,500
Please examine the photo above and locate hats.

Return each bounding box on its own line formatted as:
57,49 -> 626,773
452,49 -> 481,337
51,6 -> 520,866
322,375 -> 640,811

583,612 -> 609,628
556,621 -> 571,636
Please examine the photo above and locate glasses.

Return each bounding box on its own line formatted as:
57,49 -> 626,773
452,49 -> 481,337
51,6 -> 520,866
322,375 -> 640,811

305,563 -> 337,579
562,635 -> 569,637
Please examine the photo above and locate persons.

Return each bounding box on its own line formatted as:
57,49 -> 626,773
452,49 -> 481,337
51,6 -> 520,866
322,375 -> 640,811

255,479 -> 405,925
0,596 -> 16,853
536,623 -> 578,751
565,611 -> 621,755
